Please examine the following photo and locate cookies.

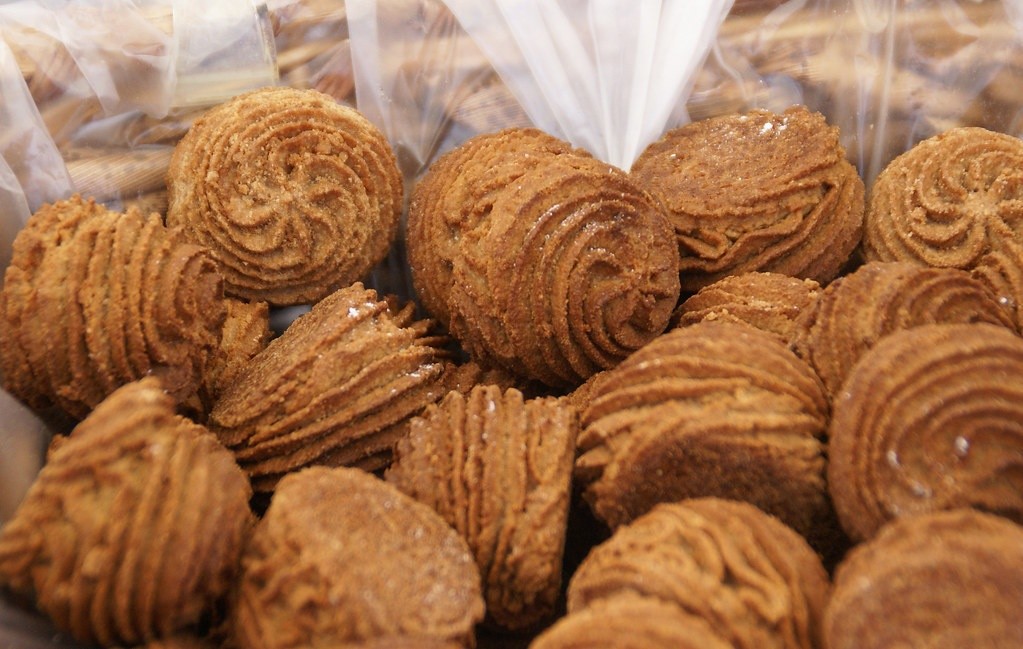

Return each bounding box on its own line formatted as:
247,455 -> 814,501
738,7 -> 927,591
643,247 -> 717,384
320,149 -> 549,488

0,0 -> 1022,212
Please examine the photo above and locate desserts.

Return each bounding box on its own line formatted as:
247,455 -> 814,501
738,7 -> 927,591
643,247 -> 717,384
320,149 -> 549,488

0,83 -> 1023,649
